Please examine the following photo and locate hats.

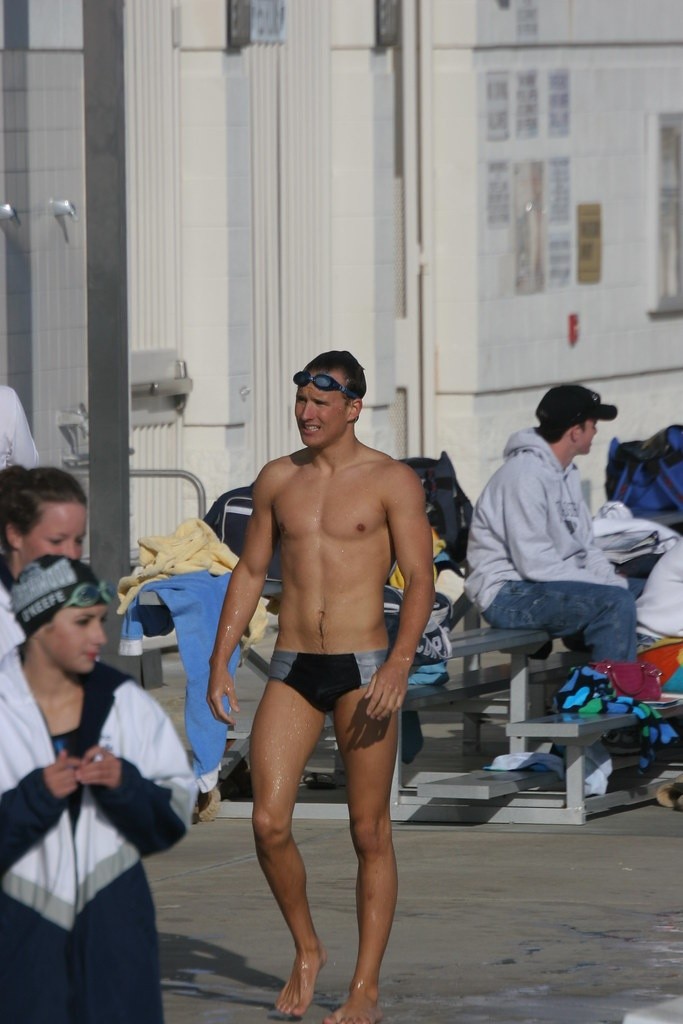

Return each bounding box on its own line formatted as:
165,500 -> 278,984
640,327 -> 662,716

535,386 -> 617,424
11,554 -> 108,635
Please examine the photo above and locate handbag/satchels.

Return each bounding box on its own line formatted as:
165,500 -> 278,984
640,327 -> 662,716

591,660 -> 662,702
202,481 -> 283,582
382,585 -> 453,685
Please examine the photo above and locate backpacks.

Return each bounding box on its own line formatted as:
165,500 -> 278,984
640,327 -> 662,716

395,451 -> 474,561
605,425 -> 683,514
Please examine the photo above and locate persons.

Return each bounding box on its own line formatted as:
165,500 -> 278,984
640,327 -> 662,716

206,352 -> 436,1024
0,554 -> 199,1024
464,385 -> 643,755
0,466 -> 87,587
0,384 -> 39,471
634,537 -> 683,811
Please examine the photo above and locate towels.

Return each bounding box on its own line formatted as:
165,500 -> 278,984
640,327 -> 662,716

0,638 -> 202,934
115,518 -> 269,794
482,664 -> 678,798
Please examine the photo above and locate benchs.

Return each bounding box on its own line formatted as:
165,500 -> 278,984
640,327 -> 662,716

139,566 -> 683,825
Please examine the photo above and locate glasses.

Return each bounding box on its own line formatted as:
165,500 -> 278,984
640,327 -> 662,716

60,578 -> 116,609
293,371 -> 358,400
